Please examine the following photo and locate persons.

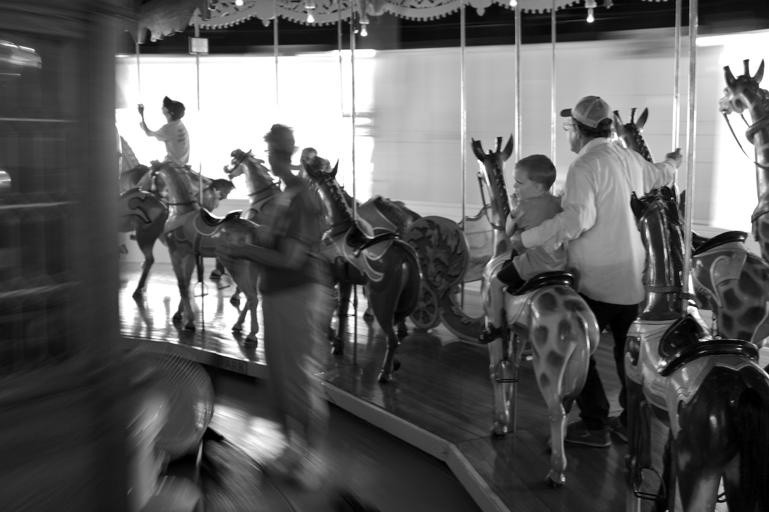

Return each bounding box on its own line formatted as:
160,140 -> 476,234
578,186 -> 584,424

220,123 -> 331,492
477,154 -> 568,345
138,95 -> 189,170
511,96 -> 688,449
288,148 -> 322,194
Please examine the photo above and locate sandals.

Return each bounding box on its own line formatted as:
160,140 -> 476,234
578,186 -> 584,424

478,322 -> 506,346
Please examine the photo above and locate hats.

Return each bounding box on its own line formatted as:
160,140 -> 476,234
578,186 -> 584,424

559,95 -> 613,130
163,95 -> 186,118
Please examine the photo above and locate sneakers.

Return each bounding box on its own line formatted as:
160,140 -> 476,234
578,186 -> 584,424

608,416 -> 630,443
564,420 -> 612,448
260,443 -> 333,494
